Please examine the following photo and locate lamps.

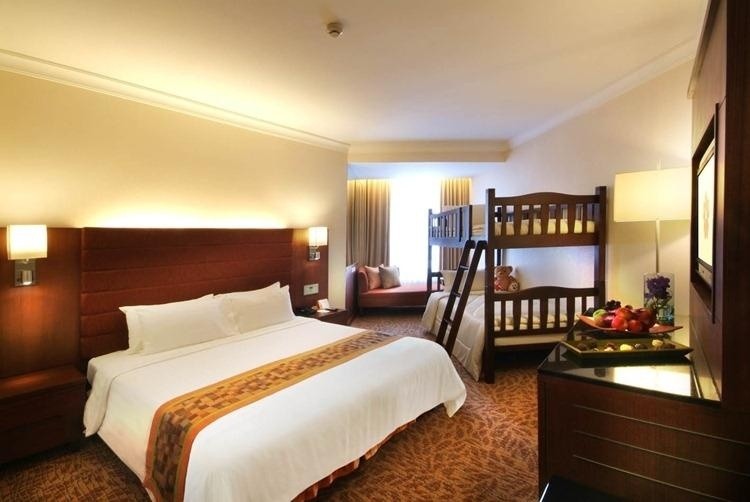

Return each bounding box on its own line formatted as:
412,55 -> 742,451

308,226 -> 328,260
6,224 -> 48,287
613,169 -> 692,274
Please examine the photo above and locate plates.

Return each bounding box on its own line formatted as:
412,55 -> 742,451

559,337 -> 694,359
576,315 -> 683,337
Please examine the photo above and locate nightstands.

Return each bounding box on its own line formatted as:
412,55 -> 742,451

309,308 -> 346,325
0,364 -> 88,467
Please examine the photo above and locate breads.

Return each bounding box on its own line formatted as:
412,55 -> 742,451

577,339 -> 674,352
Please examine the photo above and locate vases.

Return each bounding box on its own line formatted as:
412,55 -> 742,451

644,273 -> 674,325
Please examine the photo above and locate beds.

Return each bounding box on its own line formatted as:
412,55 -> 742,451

358,267 -> 444,318
428,186 -> 606,382
80,229 -> 468,502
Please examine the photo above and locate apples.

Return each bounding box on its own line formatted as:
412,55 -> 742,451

593,308 -> 653,332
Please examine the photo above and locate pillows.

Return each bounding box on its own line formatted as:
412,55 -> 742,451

214,281 -> 279,298
440,269 -> 485,295
136,297 -> 234,357
223,285 -> 295,333
119,293 -> 215,355
365,264 -> 384,290
379,266 -> 401,289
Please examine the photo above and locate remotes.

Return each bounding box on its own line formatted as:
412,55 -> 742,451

325,307 -> 338,313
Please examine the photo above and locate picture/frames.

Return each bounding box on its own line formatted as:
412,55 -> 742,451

689,103 -> 719,324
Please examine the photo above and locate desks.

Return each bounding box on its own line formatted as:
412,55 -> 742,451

536,310 -> 750,502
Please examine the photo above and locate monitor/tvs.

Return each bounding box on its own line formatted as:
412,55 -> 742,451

691,138 -> 717,290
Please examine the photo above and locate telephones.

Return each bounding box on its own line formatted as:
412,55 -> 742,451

298,308 -> 317,315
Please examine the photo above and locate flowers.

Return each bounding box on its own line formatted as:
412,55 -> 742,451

645,276 -> 671,316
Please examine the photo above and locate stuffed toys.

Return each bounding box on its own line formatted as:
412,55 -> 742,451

494,265 -> 520,293
